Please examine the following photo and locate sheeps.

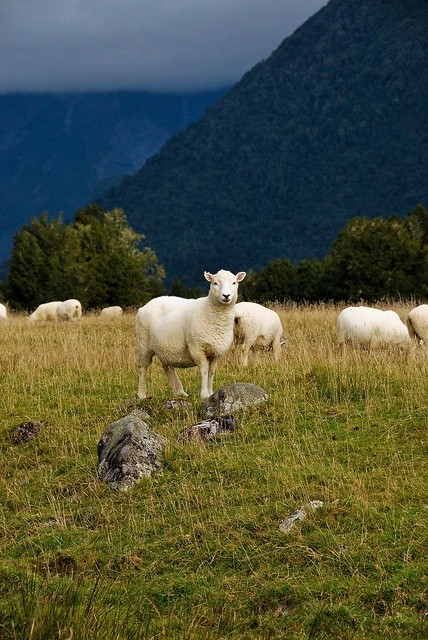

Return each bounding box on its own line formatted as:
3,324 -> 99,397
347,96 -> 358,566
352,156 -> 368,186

335,306 -> 411,352
403,303 -> 428,347
99,305 -> 123,321
56,298 -> 82,322
0,303 -> 8,320
134,268 -> 247,400
231,301 -> 287,369
25,300 -> 63,323
